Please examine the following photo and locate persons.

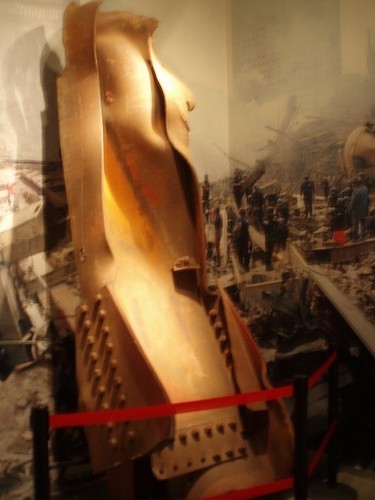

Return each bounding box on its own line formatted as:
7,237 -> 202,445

199,167 -> 375,274
